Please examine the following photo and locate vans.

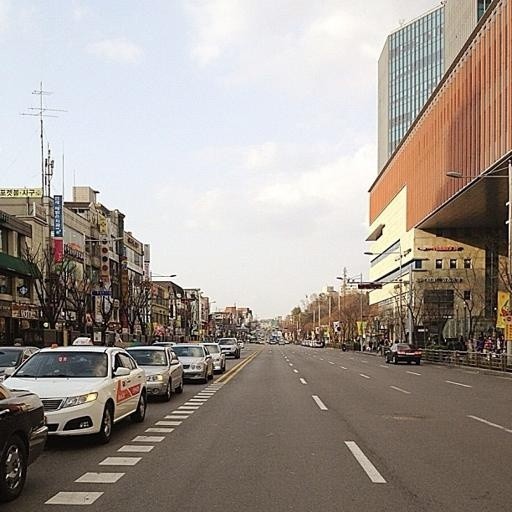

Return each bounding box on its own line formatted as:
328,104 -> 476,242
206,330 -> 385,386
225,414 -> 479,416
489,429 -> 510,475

427,334 -> 445,346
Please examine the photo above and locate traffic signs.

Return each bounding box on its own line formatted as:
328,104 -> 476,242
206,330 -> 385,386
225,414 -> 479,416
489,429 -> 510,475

358,284 -> 383,290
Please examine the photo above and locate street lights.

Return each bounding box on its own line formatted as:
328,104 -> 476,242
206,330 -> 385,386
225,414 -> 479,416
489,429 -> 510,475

337,272 -> 364,351
446,159 -> 512,289
150,272 -> 176,333
494,307 -> 497,332
306,290 -> 341,342
463,302 -> 467,333
456,304 -> 459,324
82,232 -> 124,335
364,252 -> 402,345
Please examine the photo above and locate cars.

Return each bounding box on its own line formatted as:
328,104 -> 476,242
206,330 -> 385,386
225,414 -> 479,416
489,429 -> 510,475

245,327 -> 323,349
151,337 -> 244,385
342,339 -> 354,351
1,345 -> 39,384
384,342 -> 424,366
122,346 -> 185,401
1,382 -> 49,504
0,337 -> 148,446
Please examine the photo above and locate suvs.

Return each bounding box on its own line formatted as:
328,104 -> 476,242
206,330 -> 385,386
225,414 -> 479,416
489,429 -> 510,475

446,337 -> 463,351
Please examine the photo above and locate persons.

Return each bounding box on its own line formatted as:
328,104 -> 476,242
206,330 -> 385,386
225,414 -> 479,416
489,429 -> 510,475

461,335 -> 506,360
368,335 -> 389,353
151,352 -> 163,363
87,355 -> 106,376
